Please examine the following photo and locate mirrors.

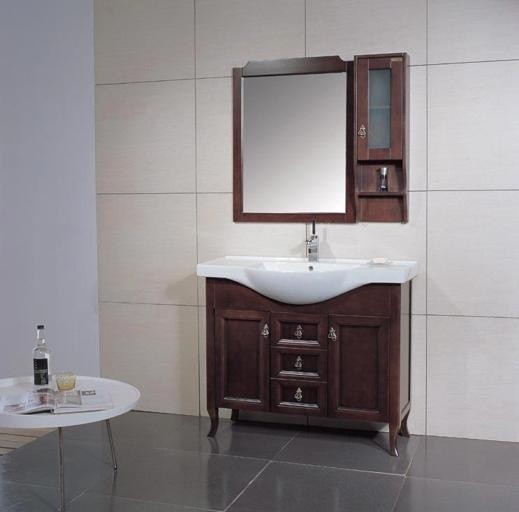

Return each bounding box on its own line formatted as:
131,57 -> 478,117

231,54 -> 354,223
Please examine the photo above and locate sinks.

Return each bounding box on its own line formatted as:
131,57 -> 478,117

245,259 -> 365,305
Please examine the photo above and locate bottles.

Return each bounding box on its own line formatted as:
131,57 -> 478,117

377,167 -> 389,192
32,324 -> 52,389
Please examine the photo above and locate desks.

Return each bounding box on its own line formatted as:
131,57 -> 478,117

0,375 -> 141,512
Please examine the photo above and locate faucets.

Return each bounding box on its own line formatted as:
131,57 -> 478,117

305,232 -> 320,262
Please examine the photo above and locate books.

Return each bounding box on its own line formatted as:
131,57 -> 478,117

1,385 -> 115,415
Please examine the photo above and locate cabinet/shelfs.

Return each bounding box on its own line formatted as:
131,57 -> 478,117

357,54 -> 411,222
206,281 -> 411,458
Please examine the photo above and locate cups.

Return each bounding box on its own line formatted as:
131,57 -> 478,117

56,368 -> 78,392
